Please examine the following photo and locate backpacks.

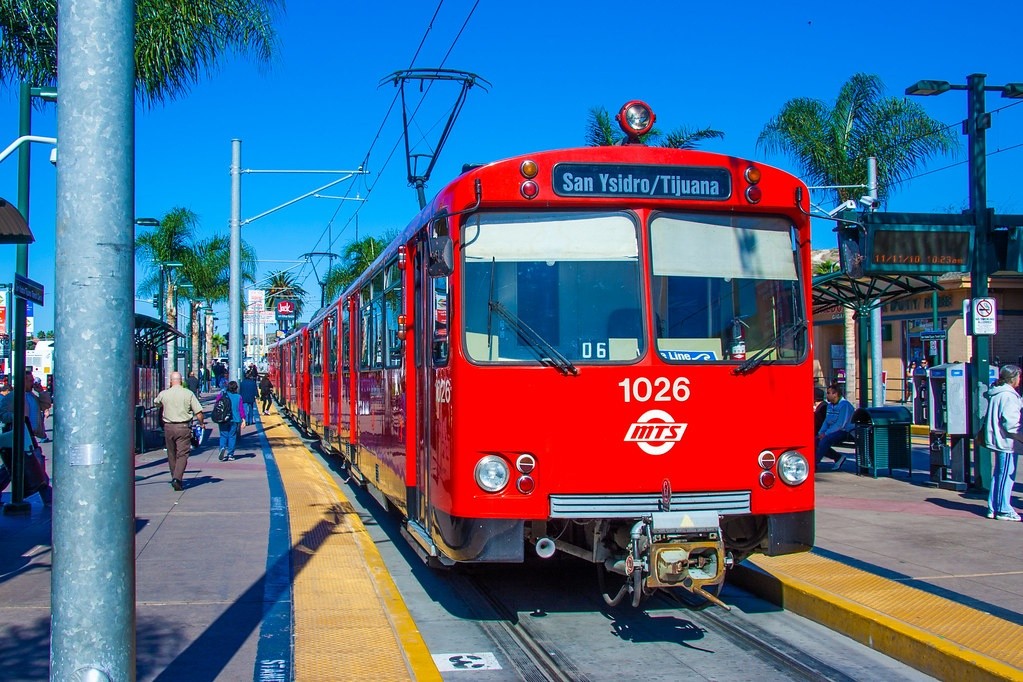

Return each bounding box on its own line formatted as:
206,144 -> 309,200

211,391 -> 233,423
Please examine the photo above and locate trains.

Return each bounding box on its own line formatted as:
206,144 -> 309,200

267,100 -> 869,611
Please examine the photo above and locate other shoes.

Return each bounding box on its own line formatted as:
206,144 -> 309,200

987,511 -> 996,518
218,448 -> 225,460
996,512 -> 1021,521
228,457 -> 236,461
262,410 -> 270,416
832,455 -> 846,470
246,422 -> 255,426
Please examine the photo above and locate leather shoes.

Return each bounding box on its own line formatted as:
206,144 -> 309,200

172,479 -> 183,491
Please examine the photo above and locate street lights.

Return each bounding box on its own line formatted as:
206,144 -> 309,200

158,261 -> 184,393
13,82 -> 58,506
173,282 -> 194,372
836,211 -> 868,407
189,297 -> 215,393
904,73 -> 1023,491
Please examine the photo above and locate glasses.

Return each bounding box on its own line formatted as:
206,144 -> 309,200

920,362 -> 926,364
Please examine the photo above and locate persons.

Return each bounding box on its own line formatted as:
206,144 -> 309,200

0,371 -> 55,507
214,381 -> 246,461
154,372 -> 205,491
905,359 -> 930,405
983,364 -> 1023,522
812,383 -> 856,470
188,362 -> 229,401
241,364 -> 274,425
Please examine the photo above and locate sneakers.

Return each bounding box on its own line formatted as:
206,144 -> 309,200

196,426 -> 205,444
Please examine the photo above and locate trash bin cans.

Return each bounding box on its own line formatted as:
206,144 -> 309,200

134,405 -> 148,455
850,406 -> 914,479
